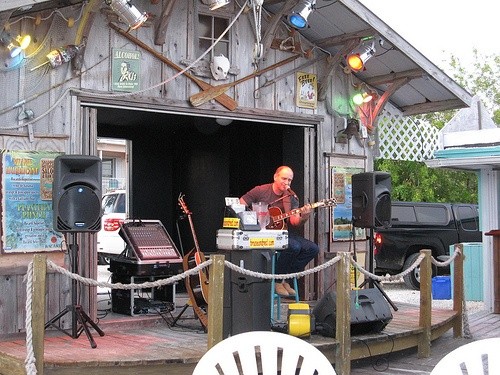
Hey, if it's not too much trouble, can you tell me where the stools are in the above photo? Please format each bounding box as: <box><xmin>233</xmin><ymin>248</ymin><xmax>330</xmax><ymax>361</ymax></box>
<box><xmin>271</xmin><ymin>252</ymin><xmax>299</xmax><ymax>326</ymax></box>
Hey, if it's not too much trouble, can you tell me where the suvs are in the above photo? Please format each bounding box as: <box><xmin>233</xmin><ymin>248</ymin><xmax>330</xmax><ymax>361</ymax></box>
<box><xmin>374</xmin><ymin>202</ymin><xmax>483</xmax><ymax>291</ymax></box>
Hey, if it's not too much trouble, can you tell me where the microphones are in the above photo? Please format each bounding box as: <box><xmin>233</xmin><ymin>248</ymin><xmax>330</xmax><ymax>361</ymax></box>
<box><xmin>285</xmin><ymin>185</ymin><xmax>301</xmax><ymax>201</ymax></box>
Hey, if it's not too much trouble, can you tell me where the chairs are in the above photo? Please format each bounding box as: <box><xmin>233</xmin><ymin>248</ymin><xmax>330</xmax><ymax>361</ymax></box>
<box><xmin>192</xmin><ymin>331</ymin><xmax>337</xmax><ymax>375</ymax></box>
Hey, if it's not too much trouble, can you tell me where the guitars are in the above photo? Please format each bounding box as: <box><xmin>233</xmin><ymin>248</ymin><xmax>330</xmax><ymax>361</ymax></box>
<box><xmin>265</xmin><ymin>198</ymin><xmax>337</xmax><ymax>230</ymax></box>
<box><xmin>177</xmin><ymin>193</ymin><xmax>209</xmax><ymax>307</ymax></box>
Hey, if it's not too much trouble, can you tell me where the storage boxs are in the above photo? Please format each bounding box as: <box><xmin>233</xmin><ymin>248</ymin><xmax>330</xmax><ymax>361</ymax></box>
<box><xmin>431</xmin><ymin>278</ymin><xmax>451</xmax><ymax>300</ymax></box>
<box><xmin>224</xmin><ymin>206</ymin><xmax>261</xmax><ymax>231</ymax></box>
<box><xmin>216</xmin><ymin>229</ymin><xmax>289</xmax><ymax>250</ymax></box>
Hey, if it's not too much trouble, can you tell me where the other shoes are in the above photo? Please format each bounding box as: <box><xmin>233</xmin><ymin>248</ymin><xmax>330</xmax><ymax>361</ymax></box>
<box><xmin>283</xmin><ymin>282</ymin><xmax>296</xmax><ymax>295</ymax></box>
<box><xmin>275</xmin><ymin>282</ymin><xmax>290</xmax><ymax>297</ymax></box>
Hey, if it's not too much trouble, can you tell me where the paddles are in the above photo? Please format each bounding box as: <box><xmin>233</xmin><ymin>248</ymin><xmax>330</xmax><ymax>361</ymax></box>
<box><xmin>189</xmin><ymin>53</ymin><xmax>304</xmax><ymax>106</ymax></box>
<box><xmin>108</xmin><ymin>22</ymin><xmax>237</xmax><ymax>110</ymax></box>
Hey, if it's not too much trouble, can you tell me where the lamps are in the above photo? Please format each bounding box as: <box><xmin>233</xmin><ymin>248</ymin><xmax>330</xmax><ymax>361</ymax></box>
<box><xmin>346</xmin><ymin>36</ymin><xmax>392</xmax><ymax>72</ymax></box>
<box><xmin>286</xmin><ymin>0</ymin><xmax>317</xmax><ymax>29</ymax></box>
<box><xmin>353</xmin><ymin>83</ymin><xmax>379</xmax><ymax>104</ymax></box>
<box><xmin>206</xmin><ymin>0</ymin><xmax>230</xmax><ymax>11</ymax></box>
<box><xmin>105</xmin><ymin>0</ymin><xmax>150</xmax><ymax>30</ymax></box>
<box><xmin>0</xmin><ymin>32</ymin><xmax>30</xmax><ymax>58</ymax></box>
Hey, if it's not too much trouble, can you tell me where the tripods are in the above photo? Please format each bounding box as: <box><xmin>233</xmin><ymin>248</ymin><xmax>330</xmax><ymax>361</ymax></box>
<box><xmin>358</xmin><ymin>227</ymin><xmax>398</xmax><ymax>312</ymax></box>
<box><xmin>25</xmin><ymin>233</ymin><xmax>106</xmax><ymax>349</ymax></box>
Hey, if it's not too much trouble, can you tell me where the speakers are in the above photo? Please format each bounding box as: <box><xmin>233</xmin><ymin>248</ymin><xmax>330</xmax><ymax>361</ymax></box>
<box><xmin>52</xmin><ymin>153</ymin><xmax>103</xmax><ymax>233</ymax></box>
<box><xmin>350</xmin><ymin>170</ymin><xmax>393</xmax><ymax>230</ymax></box>
<box><xmin>312</xmin><ymin>287</ymin><xmax>394</xmax><ymax>335</ymax></box>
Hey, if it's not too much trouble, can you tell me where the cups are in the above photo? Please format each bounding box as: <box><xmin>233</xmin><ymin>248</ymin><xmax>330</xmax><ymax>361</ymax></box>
<box><xmin>251</xmin><ymin>202</ymin><xmax>270</xmax><ymax>231</ymax></box>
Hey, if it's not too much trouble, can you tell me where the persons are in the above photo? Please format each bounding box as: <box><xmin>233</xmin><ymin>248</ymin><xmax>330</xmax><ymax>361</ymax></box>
<box><xmin>240</xmin><ymin>166</ymin><xmax>320</xmax><ymax>296</ymax></box>
